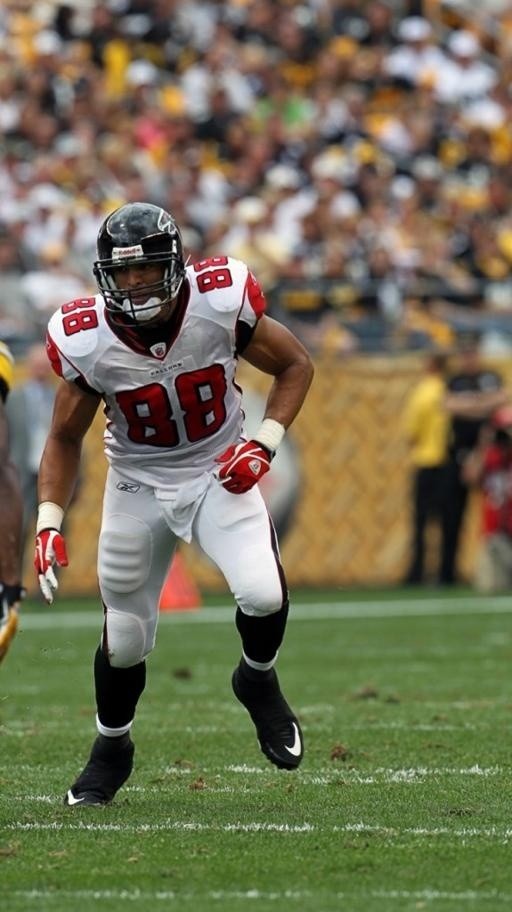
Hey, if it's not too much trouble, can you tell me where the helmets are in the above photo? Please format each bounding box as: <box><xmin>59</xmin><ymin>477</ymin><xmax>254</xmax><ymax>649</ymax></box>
<box><xmin>93</xmin><ymin>200</ymin><xmax>187</xmax><ymax>330</ymax></box>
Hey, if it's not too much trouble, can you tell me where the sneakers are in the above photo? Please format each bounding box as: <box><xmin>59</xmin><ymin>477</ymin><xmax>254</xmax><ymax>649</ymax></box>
<box><xmin>63</xmin><ymin>734</ymin><xmax>136</xmax><ymax>810</ymax></box>
<box><xmin>231</xmin><ymin>659</ymin><xmax>304</xmax><ymax>772</ymax></box>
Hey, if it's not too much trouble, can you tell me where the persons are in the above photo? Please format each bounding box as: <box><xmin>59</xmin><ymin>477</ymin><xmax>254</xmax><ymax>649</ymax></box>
<box><xmin>32</xmin><ymin>200</ymin><xmax>315</xmax><ymax>806</ymax></box>
<box><xmin>0</xmin><ymin>1</ymin><xmax>510</xmax><ymax>660</ymax></box>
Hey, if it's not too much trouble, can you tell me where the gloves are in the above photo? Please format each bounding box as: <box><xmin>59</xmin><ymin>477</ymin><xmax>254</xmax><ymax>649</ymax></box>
<box><xmin>31</xmin><ymin>527</ymin><xmax>70</xmax><ymax>606</ymax></box>
<box><xmin>209</xmin><ymin>439</ymin><xmax>272</xmax><ymax>495</ymax></box>
